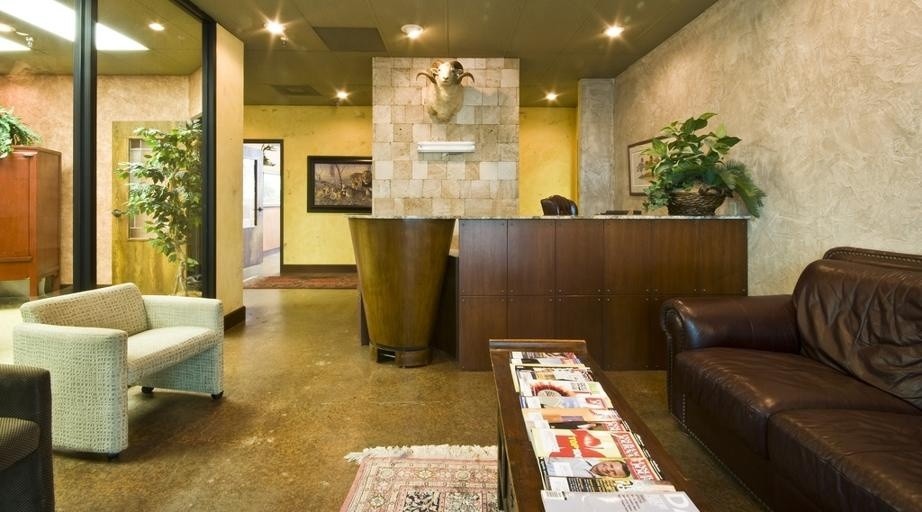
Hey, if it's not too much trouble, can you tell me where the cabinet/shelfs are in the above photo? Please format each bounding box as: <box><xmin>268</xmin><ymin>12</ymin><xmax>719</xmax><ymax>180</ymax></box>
<box><xmin>1</xmin><ymin>144</ymin><xmax>62</xmax><ymax>303</ymax></box>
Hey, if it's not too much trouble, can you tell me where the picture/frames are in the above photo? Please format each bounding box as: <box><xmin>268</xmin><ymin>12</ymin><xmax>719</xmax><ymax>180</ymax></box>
<box><xmin>306</xmin><ymin>155</ymin><xmax>373</xmax><ymax>215</ymax></box>
<box><xmin>628</xmin><ymin>135</ymin><xmax>679</xmax><ymax>197</ymax></box>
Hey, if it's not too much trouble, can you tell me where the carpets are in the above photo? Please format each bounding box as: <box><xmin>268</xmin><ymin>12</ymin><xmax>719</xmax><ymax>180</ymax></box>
<box><xmin>335</xmin><ymin>443</ymin><xmax>500</xmax><ymax>512</ymax></box>
<box><xmin>243</xmin><ymin>273</ymin><xmax>359</xmax><ymax>290</ymax></box>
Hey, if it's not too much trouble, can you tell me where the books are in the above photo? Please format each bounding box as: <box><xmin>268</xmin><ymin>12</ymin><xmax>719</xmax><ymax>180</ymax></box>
<box><xmin>505</xmin><ymin>347</ymin><xmax>700</xmax><ymax>511</ymax></box>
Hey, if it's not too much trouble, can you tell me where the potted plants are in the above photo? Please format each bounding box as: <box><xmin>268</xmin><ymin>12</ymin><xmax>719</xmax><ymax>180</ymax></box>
<box><xmin>640</xmin><ymin>113</ymin><xmax>767</xmax><ymax>218</ymax></box>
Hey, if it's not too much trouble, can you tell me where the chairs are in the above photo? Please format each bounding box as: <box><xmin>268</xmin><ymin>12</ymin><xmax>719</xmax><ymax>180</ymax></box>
<box><xmin>541</xmin><ymin>194</ymin><xmax>578</xmax><ymax>216</ymax></box>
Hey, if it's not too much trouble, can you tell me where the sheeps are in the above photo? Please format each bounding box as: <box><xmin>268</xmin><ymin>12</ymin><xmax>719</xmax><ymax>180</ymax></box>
<box><xmin>414</xmin><ymin>57</ymin><xmax>473</xmax><ymax>125</ymax></box>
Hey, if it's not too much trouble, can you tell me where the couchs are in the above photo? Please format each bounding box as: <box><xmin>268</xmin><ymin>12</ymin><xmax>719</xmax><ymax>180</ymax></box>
<box><xmin>657</xmin><ymin>247</ymin><xmax>921</xmax><ymax>512</ymax></box>
<box><xmin>12</xmin><ymin>281</ymin><xmax>225</xmax><ymax>457</ymax></box>
<box><xmin>0</xmin><ymin>361</ymin><xmax>55</xmax><ymax>512</ymax></box>
